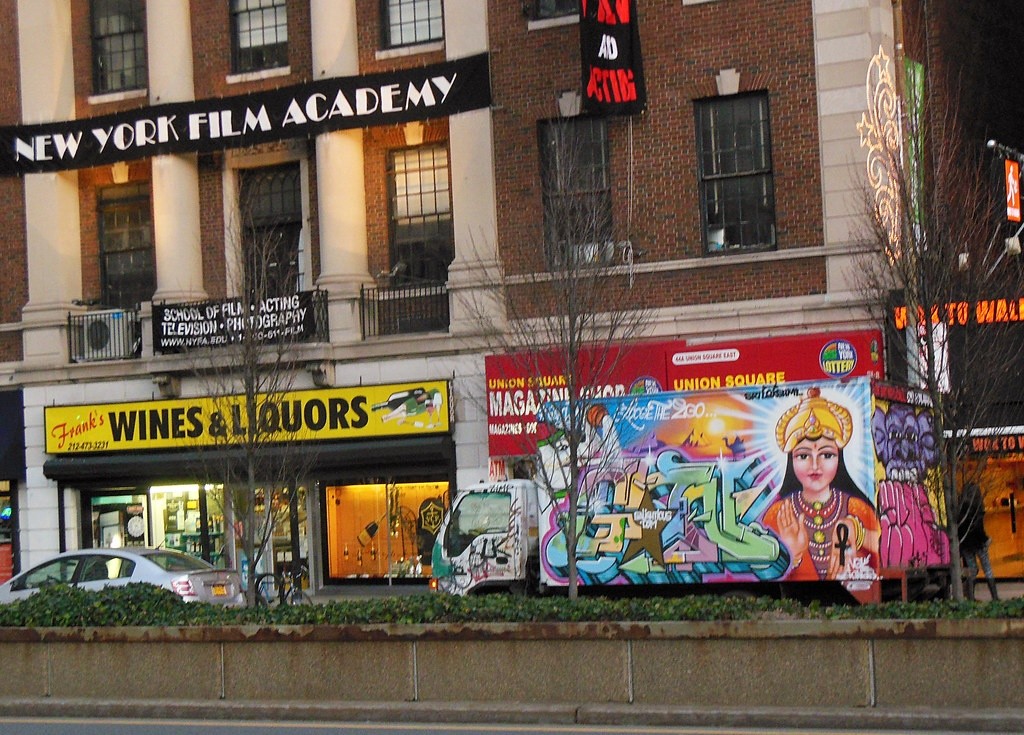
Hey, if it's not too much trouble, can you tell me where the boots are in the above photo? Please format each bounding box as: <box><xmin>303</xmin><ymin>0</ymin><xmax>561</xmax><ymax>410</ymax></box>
<box><xmin>989</xmin><ymin>579</ymin><xmax>998</xmax><ymax>600</ymax></box>
<box><xmin>968</xmin><ymin>580</ymin><xmax>975</xmax><ymax>601</ymax></box>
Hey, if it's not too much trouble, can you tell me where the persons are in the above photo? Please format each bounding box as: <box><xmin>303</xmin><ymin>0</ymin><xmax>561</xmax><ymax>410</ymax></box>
<box><xmin>956</xmin><ymin>482</ymin><xmax>1000</xmax><ymax>602</ymax></box>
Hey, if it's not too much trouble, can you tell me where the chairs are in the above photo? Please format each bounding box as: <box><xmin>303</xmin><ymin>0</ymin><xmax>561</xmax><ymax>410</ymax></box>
<box><xmin>125</xmin><ymin>565</ymin><xmax>134</xmax><ymax>577</ymax></box>
<box><xmin>92</xmin><ymin>562</ymin><xmax>108</xmax><ymax>579</ymax></box>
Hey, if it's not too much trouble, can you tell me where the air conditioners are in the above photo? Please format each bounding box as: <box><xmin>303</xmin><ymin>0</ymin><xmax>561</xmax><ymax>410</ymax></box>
<box><xmin>84</xmin><ymin>309</ymin><xmax>131</xmax><ymax>359</ymax></box>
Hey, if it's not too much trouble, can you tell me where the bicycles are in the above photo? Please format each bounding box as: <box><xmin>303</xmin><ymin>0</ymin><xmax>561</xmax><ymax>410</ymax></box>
<box><xmin>253</xmin><ymin>562</ymin><xmax>316</xmax><ymax>608</ymax></box>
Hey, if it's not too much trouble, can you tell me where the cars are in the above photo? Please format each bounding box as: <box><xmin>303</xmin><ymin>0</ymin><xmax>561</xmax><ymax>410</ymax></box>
<box><xmin>2</xmin><ymin>546</ymin><xmax>248</xmax><ymax>613</ymax></box>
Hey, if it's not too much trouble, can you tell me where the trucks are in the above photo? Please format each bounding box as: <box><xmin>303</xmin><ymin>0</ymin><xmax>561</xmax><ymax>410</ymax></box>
<box><xmin>429</xmin><ymin>372</ymin><xmax>952</xmax><ymax>608</ymax></box>
